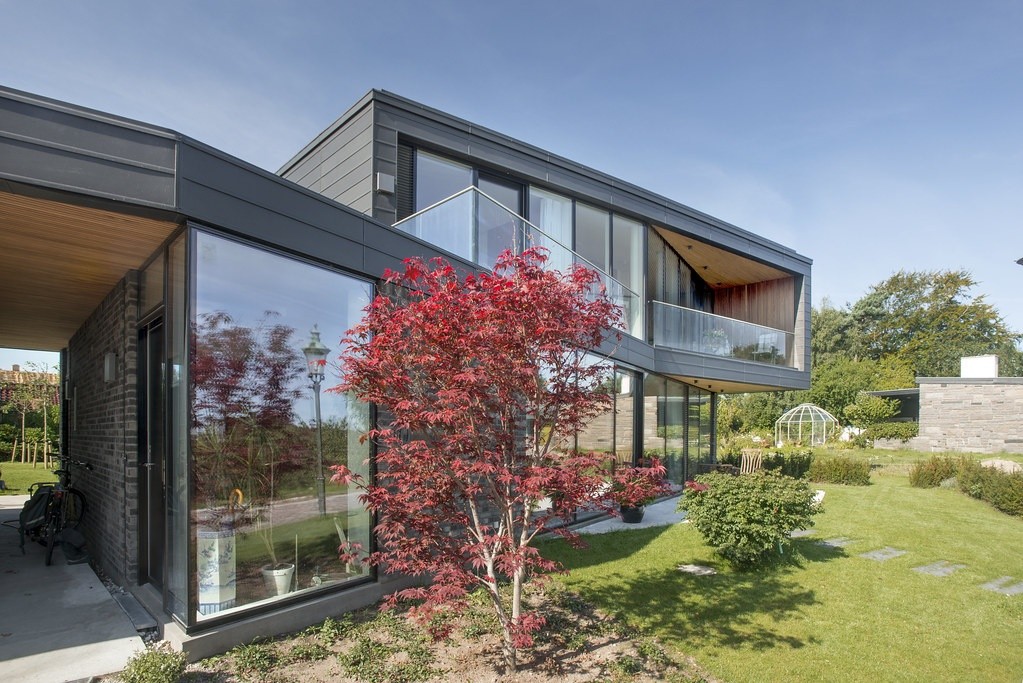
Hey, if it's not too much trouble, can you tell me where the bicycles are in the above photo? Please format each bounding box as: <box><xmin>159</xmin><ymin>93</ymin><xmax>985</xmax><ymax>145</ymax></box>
<box><xmin>31</xmin><ymin>452</ymin><xmax>95</xmax><ymax>566</ymax></box>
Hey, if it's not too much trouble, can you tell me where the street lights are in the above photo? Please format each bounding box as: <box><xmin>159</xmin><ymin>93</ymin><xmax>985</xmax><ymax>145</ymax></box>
<box><xmin>300</xmin><ymin>322</ymin><xmax>332</xmax><ymax>516</ymax></box>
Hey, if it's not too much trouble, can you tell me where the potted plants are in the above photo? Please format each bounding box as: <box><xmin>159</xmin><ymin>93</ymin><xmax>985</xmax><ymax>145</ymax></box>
<box><xmin>192</xmin><ymin>422</ymin><xmax>247</xmax><ymax>614</ymax></box>
<box><xmin>231</xmin><ymin>401</ymin><xmax>295</xmax><ymax>596</ymax></box>
<box><xmin>614</xmin><ymin>474</ymin><xmax>656</xmax><ymax>523</ymax></box>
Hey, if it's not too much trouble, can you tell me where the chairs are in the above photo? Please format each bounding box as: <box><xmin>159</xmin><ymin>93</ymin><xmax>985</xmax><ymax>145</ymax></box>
<box><xmin>751</xmin><ymin>332</ymin><xmax>779</xmax><ymax>367</ymax></box>
